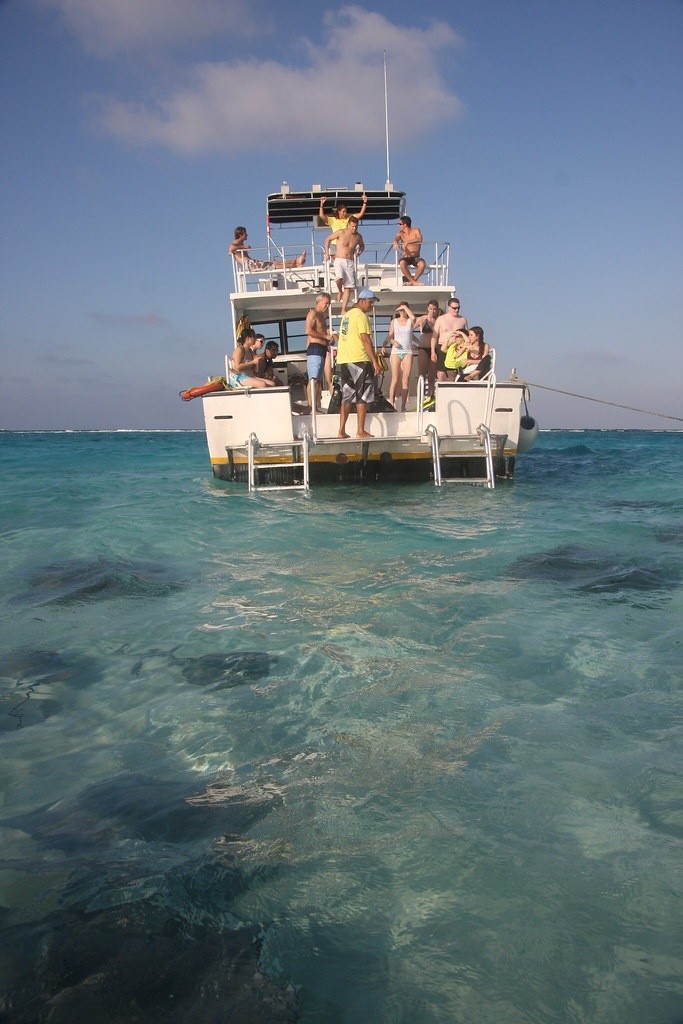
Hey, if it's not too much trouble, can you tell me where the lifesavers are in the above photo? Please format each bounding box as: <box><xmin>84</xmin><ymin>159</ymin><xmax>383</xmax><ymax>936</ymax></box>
<box><xmin>288</xmin><ymin>372</ymin><xmax>312</xmax><ymax>414</ymax></box>
<box><xmin>179</xmin><ymin>380</ymin><xmax>226</xmax><ymax>400</ymax></box>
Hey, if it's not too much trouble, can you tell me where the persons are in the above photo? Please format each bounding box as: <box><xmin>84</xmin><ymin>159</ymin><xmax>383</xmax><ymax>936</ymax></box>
<box><xmin>394</xmin><ymin>216</ymin><xmax>426</xmax><ymax>285</ymax></box>
<box><xmin>382</xmin><ymin>331</ymin><xmax>420</xmax><ymax>402</ymax></box>
<box><xmin>412</xmin><ymin>300</ymin><xmax>441</xmax><ymax>398</ymax></box>
<box><xmin>336</xmin><ymin>289</ymin><xmax>381</xmax><ymax>438</ymax></box>
<box><xmin>229</xmin><ymin>227</ymin><xmax>305</xmax><ymax>272</ymax></box>
<box><xmin>324</xmin><ymin>216</ymin><xmax>364</xmax><ymax>314</ymax></box>
<box><xmin>431</xmin><ymin>298</ymin><xmax>468</xmax><ymax>382</ymax></box>
<box><xmin>231</xmin><ymin>328</ymin><xmax>311</xmax><ymax>414</ymax></box>
<box><xmin>389</xmin><ymin>302</ymin><xmax>416</xmax><ymax>411</ymax></box>
<box><xmin>305</xmin><ymin>292</ymin><xmax>340</xmax><ymax>414</ymax></box>
<box><xmin>441</xmin><ymin>326</ymin><xmax>491</xmax><ymax>382</ymax></box>
<box><xmin>319</xmin><ymin>195</ymin><xmax>367</xmax><ymax>260</ymax></box>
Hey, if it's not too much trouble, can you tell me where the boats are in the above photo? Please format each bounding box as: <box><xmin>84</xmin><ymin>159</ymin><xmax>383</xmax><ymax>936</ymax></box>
<box><xmin>179</xmin><ymin>49</ymin><xmax>538</xmax><ymax>497</ymax></box>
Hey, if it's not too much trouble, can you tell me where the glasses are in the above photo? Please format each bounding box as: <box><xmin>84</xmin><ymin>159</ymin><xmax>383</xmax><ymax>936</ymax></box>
<box><xmin>398</xmin><ymin>223</ymin><xmax>402</xmax><ymax>226</ymax></box>
<box><xmin>256</xmin><ymin>338</ymin><xmax>264</xmax><ymax>343</ymax></box>
<box><xmin>450</xmin><ymin>306</ymin><xmax>460</xmax><ymax>310</ymax></box>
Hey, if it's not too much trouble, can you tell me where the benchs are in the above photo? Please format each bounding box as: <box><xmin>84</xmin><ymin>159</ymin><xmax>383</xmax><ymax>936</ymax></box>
<box><xmin>224</xmin><ymin>355</ymin><xmax>255</xmax><ymax>389</ymax></box>
<box><xmin>453</xmin><ymin>348</ymin><xmax>496</xmax><ymax>381</ymax></box>
<box><xmin>364</xmin><ymin>264</ymin><xmax>446</xmax><ymax>286</ymax></box>
<box><xmin>237</xmin><ymin>265</ymin><xmax>326</xmax><ymax>293</ymax></box>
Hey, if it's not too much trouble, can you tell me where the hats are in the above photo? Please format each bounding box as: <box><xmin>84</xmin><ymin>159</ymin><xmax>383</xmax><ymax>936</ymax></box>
<box><xmin>358</xmin><ymin>289</ymin><xmax>379</xmax><ymax>299</ymax></box>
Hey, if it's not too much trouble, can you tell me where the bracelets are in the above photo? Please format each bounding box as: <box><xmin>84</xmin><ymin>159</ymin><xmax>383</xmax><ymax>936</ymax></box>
<box><xmin>446</xmin><ymin>340</ymin><xmax>449</xmax><ymax>342</ymax></box>
<box><xmin>364</xmin><ymin>202</ymin><xmax>367</xmax><ymax>204</ymax></box>
<box><xmin>320</xmin><ymin>207</ymin><xmax>323</xmax><ymax>208</ymax></box>
